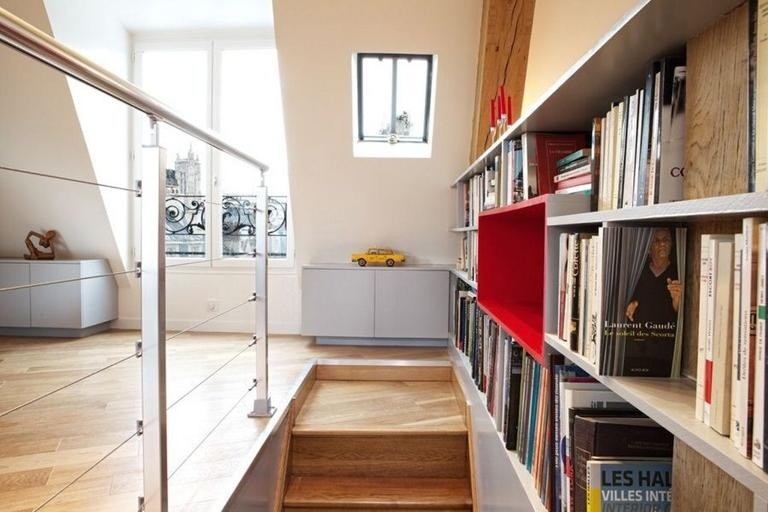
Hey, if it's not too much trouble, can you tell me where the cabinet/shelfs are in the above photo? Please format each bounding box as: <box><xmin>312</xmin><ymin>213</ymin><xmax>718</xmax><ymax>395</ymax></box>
<box><xmin>298</xmin><ymin>262</ymin><xmax>456</xmax><ymax>347</ymax></box>
<box><xmin>444</xmin><ymin>0</ymin><xmax>766</xmax><ymax>512</ymax></box>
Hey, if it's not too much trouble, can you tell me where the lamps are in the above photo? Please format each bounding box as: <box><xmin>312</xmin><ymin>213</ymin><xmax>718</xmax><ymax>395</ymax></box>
<box><xmin>387</xmin><ymin>135</ymin><xmax>398</xmax><ymax>144</ymax></box>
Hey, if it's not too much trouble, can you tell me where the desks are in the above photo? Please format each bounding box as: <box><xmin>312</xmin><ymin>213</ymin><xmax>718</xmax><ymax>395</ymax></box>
<box><xmin>0</xmin><ymin>259</ymin><xmax>119</xmax><ymax>338</ymax></box>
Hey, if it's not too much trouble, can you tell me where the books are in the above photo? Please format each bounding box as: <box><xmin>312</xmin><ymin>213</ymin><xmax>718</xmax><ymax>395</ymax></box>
<box><xmin>461</xmin><ymin>131</ymin><xmax>591</xmax><ymax>282</ymax></box>
<box><xmin>455</xmin><ymin>276</ymin><xmax>553</xmax><ymax>511</ymax></box>
<box><xmin>547</xmin><ymin>353</ymin><xmax>674</xmax><ymax>512</ymax></box>
<box><xmin>590</xmin><ymin>57</ymin><xmax>687</xmax><ymax>212</ymax></box>
<box><xmin>557</xmin><ymin>221</ymin><xmax>686</xmax><ymax>376</ymax></box>
<box><xmin>696</xmin><ymin>216</ymin><xmax>768</xmax><ymax>473</ymax></box>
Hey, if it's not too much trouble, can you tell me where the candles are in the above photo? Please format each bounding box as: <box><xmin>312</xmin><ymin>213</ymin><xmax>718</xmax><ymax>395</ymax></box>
<box><xmin>489</xmin><ymin>85</ymin><xmax>512</xmax><ymax>128</ymax></box>
